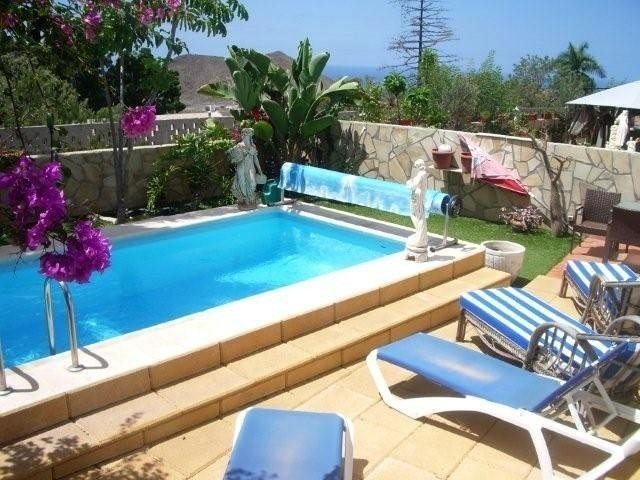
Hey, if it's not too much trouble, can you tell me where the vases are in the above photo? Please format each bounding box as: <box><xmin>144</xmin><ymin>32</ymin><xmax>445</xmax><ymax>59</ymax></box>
<box><xmin>480</xmin><ymin>236</ymin><xmax>526</xmax><ymax>282</ymax></box>
<box><xmin>458</xmin><ymin>152</ymin><xmax>472</xmax><ymax>174</ymax></box>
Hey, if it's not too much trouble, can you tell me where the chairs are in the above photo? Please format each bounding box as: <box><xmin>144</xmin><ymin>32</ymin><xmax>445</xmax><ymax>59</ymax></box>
<box><xmin>363</xmin><ymin>327</ymin><xmax>638</xmax><ymax>480</ymax></box>
<box><xmin>556</xmin><ymin>258</ymin><xmax>640</xmax><ymax>333</ymax></box>
<box><xmin>568</xmin><ymin>188</ymin><xmax>622</xmax><ymax>256</ymax></box>
<box><xmin>452</xmin><ymin>284</ymin><xmax>640</xmax><ymax>402</ymax></box>
<box><xmin>218</xmin><ymin>403</ymin><xmax>358</xmax><ymax>479</ymax></box>
<box><xmin>603</xmin><ymin>202</ymin><xmax>639</xmax><ymax>268</ymax></box>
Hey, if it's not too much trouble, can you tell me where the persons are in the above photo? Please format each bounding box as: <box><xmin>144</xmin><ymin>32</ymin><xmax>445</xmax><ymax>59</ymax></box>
<box><xmin>406</xmin><ymin>159</ymin><xmax>432</xmax><ymax>247</ymax></box>
<box><xmin>227</xmin><ymin>128</ymin><xmax>261</xmax><ymax>204</ymax></box>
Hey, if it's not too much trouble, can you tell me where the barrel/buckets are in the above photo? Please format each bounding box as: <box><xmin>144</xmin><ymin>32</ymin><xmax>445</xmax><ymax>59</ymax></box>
<box><xmin>262</xmin><ymin>176</ymin><xmax>281</xmax><ymax>205</ymax></box>
<box><xmin>461</xmin><ymin>152</ymin><xmax>472</xmax><ymax>172</ymax></box>
<box><xmin>481</xmin><ymin>240</ymin><xmax>525</xmax><ymax>284</ymax></box>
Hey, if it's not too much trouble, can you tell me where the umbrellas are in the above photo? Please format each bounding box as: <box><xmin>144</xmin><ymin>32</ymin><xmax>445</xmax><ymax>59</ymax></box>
<box><xmin>565</xmin><ymin>80</ymin><xmax>640</xmax><ymax>110</ymax></box>
